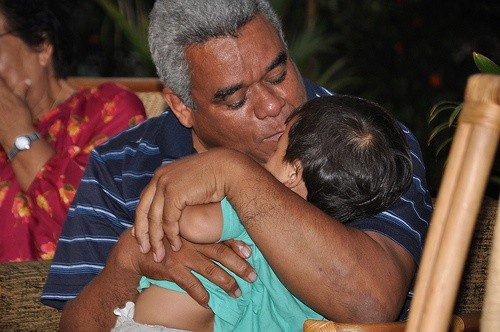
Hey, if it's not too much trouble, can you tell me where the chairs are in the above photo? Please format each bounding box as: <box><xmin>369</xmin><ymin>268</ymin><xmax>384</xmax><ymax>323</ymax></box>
<box><xmin>0</xmin><ymin>199</ymin><xmax>500</xmax><ymax>332</ymax></box>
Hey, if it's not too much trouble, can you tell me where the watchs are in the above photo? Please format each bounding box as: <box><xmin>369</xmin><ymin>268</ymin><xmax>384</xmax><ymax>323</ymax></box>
<box><xmin>8</xmin><ymin>132</ymin><xmax>40</xmax><ymax>160</ymax></box>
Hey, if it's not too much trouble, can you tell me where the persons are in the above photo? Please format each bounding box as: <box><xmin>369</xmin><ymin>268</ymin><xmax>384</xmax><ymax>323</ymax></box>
<box><xmin>38</xmin><ymin>0</ymin><xmax>432</xmax><ymax>332</ymax></box>
<box><xmin>111</xmin><ymin>94</ymin><xmax>415</xmax><ymax>331</ymax></box>
<box><xmin>0</xmin><ymin>0</ymin><xmax>146</xmax><ymax>263</ymax></box>
